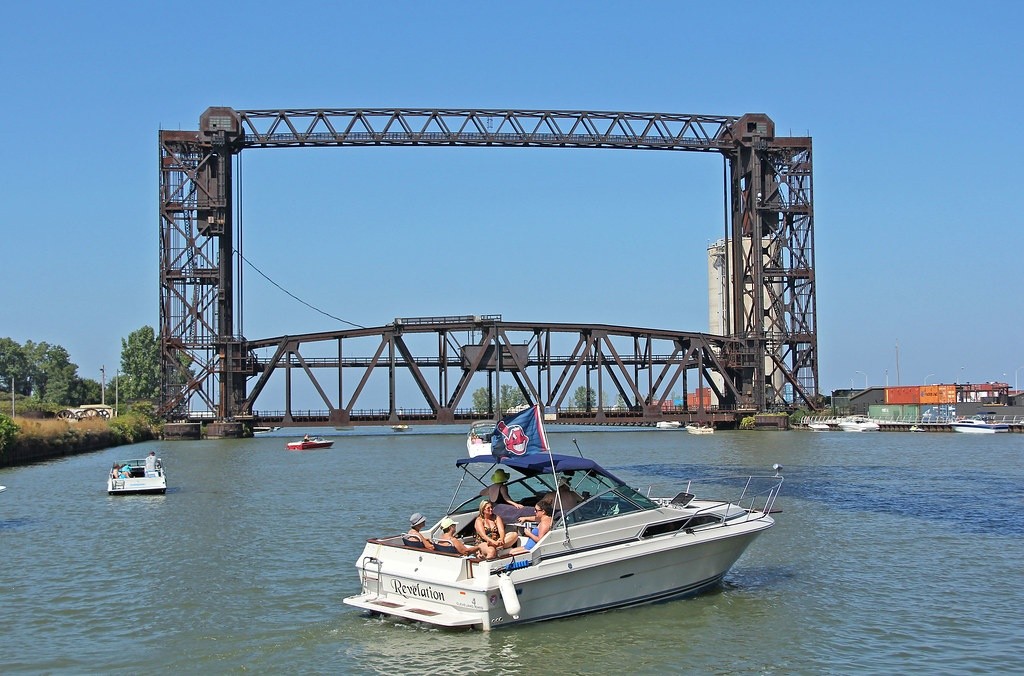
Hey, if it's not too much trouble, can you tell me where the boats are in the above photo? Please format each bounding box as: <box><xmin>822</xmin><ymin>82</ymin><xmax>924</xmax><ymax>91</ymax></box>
<box><xmin>389</xmin><ymin>424</ymin><xmax>413</xmax><ymax>432</ymax></box>
<box><xmin>808</xmin><ymin>424</ymin><xmax>830</xmax><ymax>432</ymax></box>
<box><xmin>837</xmin><ymin>414</ymin><xmax>881</xmax><ymax>433</ymax></box>
<box><xmin>947</xmin><ymin>419</ymin><xmax>1010</xmax><ymax>433</ymax></box>
<box><xmin>656</xmin><ymin>421</ymin><xmax>682</xmax><ymax>429</ymax></box>
<box><xmin>341</xmin><ymin>395</ymin><xmax>785</xmax><ymax>630</ymax></box>
<box><xmin>909</xmin><ymin>424</ymin><xmax>926</xmax><ymax>432</ymax></box>
<box><xmin>106</xmin><ymin>458</ymin><xmax>167</xmax><ymax>495</ymax></box>
<box><xmin>287</xmin><ymin>436</ymin><xmax>335</xmax><ymax>450</ymax></box>
<box><xmin>466</xmin><ymin>420</ymin><xmax>499</xmax><ymax>459</ymax></box>
<box><xmin>684</xmin><ymin>425</ymin><xmax>715</xmax><ymax>435</ymax></box>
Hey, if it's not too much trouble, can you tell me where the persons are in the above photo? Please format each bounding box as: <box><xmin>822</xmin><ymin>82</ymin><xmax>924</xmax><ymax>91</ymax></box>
<box><xmin>408</xmin><ymin>513</ymin><xmax>434</xmax><ymax>548</ymax></box>
<box><xmin>145</xmin><ymin>451</ymin><xmax>159</xmax><ymax>472</ymax></box>
<box><xmin>304</xmin><ymin>434</ymin><xmax>311</xmax><ymax>442</ymax></box>
<box><xmin>480</xmin><ymin>469</ymin><xmax>524</xmax><ymax>509</ymax></box>
<box><xmin>439</xmin><ymin>518</ymin><xmax>478</xmax><ymax>554</ymax></box>
<box><xmin>475</xmin><ymin>500</ymin><xmax>518</xmax><ymax>558</ymax></box>
<box><xmin>509</xmin><ymin>501</ymin><xmax>553</xmax><ymax>554</ymax></box>
<box><xmin>542</xmin><ymin>476</ymin><xmax>582</xmax><ymax>514</ymax></box>
<box><xmin>113</xmin><ymin>464</ymin><xmax>130</xmax><ymax>478</ymax></box>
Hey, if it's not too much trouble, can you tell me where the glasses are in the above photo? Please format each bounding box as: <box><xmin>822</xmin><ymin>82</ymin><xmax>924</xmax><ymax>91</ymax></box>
<box><xmin>484</xmin><ymin>506</ymin><xmax>493</xmax><ymax>510</ymax></box>
<box><xmin>534</xmin><ymin>509</ymin><xmax>543</xmax><ymax>513</ymax></box>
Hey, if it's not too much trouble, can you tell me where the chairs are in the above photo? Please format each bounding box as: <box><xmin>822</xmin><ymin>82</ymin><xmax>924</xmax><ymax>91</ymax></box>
<box><xmin>401</xmin><ymin>532</ymin><xmax>426</xmax><ymax>548</ymax></box>
<box><xmin>147</xmin><ymin>471</ymin><xmax>159</xmax><ymax>478</ymax></box>
<box><xmin>432</xmin><ymin>538</ymin><xmax>459</xmax><ymax>554</ymax></box>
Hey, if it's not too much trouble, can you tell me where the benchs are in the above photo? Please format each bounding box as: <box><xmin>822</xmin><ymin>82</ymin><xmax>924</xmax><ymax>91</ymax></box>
<box><xmin>492</xmin><ymin>504</ymin><xmax>569</xmax><ymax>523</ymax></box>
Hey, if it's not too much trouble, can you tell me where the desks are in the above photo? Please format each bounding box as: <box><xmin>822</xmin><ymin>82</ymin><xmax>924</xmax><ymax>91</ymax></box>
<box><xmin>505</xmin><ymin>521</ymin><xmax>540</xmax><ymax>536</ymax></box>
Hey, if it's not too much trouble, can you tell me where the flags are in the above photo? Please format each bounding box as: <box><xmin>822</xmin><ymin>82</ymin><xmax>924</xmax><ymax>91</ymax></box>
<box><xmin>491</xmin><ymin>405</ymin><xmax>547</xmax><ymax>460</ymax></box>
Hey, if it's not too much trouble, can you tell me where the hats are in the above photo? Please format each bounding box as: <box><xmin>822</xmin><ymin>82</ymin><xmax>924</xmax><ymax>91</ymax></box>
<box><xmin>409</xmin><ymin>513</ymin><xmax>427</xmax><ymax>526</ymax></box>
<box><xmin>441</xmin><ymin>518</ymin><xmax>459</xmax><ymax>529</ymax></box>
<box><xmin>556</xmin><ymin>476</ymin><xmax>572</xmax><ymax>487</ymax></box>
<box><xmin>491</xmin><ymin>469</ymin><xmax>510</xmax><ymax>483</ymax></box>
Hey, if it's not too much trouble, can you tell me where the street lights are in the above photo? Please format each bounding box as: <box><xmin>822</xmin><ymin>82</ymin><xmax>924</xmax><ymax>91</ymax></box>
<box><xmin>1015</xmin><ymin>366</ymin><xmax>1024</xmax><ymax>395</ymax></box>
<box><xmin>855</xmin><ymin>371</ymin><xmax>868</xmax><ymax>388</ymax></box>
<box><xmin>924</xmin><ymin>373</ymin><xmax>936</xmax><ymax>386</ymax></box>
